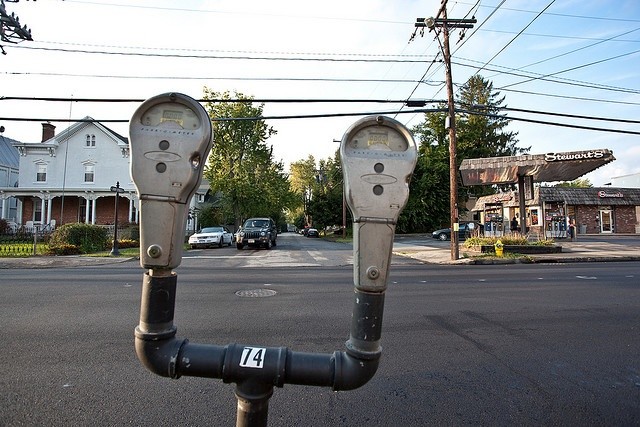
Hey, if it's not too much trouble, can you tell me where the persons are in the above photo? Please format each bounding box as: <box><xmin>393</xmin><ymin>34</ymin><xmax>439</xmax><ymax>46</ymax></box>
<box><xmin>511</xmin><ymin>217</ymin><xmax>518</xmax><ymax>230</ymax></box>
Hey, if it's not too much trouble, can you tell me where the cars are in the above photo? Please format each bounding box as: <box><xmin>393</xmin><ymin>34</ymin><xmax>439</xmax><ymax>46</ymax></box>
<box><xmin>432</xmin><ymin>220</ymin><xmax>484</xmax><ymax>241</ymax></box>
<box><xmin>306</xmin><ymin>228</ymin><xmax>320</xmax><ymax>238</ymax></box>
<box><xmin>187</xmin><ymin>226</ymin><xmax>234</xmax><ymax>250</ymax></box>
<box><xmin>235</xmin><ymin>217</ymin><xmax>277</xmax><ymax>250</ymax></box>
